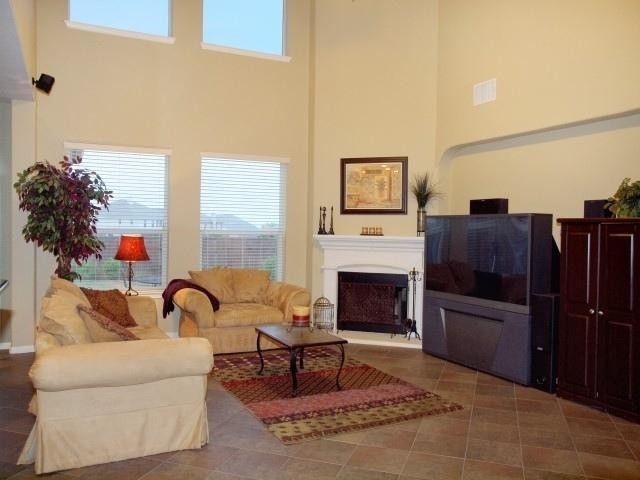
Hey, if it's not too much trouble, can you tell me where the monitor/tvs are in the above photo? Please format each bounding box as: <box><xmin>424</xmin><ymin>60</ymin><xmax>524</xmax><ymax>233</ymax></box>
<box><xmin>423</xmin><ymin>213</ymin><xmax>561</xmax><ymax>314</ymax></box>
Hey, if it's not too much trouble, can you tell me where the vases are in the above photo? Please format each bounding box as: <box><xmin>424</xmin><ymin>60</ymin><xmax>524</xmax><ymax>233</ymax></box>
<box><xmin>416</xmin><ymin>206</ymin><xmax>427</xmax><ymax>236</ymax></box>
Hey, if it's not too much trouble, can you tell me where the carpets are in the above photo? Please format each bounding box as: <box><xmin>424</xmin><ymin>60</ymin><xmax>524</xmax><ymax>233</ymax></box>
<box><xmin>209</xmin><ymin>345</ymin><xmax>464</xmax><ymax>445</ymax></box>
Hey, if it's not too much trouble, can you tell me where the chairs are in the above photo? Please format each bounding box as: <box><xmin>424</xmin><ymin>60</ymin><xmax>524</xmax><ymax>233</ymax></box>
<box><xmin>171</xmin><ymin>274</ymin><xmax>310</xmax><ymax>356</ymax></box>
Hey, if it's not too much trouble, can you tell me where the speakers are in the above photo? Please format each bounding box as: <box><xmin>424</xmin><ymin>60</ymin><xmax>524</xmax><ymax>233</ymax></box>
<box><xmin>470</xmin><ymin>199</ymin><xmax>509</xmax><ymax>214</ymax></box>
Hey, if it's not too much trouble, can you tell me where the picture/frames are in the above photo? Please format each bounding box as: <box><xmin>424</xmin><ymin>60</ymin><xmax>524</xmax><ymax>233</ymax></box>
<box><xmin>339</xmin><ymin>157</ymin><xmax>407</xmax><ymax>214</ymax></box>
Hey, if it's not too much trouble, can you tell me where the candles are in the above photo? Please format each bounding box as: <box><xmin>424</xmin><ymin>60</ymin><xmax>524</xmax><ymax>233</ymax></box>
<box><xmin>319</xmin><ymin>206</ymin><xmax>333</xmax><ymax>230</ymax></box>
<box><xmin>362</xmin><ymin>227</ymin><xmax>382</xmax><ymax>234</ymax></box>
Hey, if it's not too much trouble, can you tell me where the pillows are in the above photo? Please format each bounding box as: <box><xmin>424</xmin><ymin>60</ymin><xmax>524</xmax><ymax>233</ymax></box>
<box><xmin>188</xmin><ymin>267</ymin><xmax>271</xmax><ymax>303</ymax></box>
<box><xmin>36</xmin><ymin>274</ymin><xmax>142</xmax><ymax>346</ymax></box>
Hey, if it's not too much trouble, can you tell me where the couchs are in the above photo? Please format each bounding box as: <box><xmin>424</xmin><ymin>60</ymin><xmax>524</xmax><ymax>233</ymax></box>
<box><xmin>16</xmin><ymin>295</ymin><xmax>214</xmax><ymax>475</ymax></box>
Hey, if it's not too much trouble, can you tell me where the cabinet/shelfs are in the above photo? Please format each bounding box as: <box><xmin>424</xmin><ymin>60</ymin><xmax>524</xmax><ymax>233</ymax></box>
<box><xmin>556</xmin><ymin>217</ymin><xmax>637</xmax><ymax>424</ymax></box>
<box><xmin>422</xmin><ymin>296</ymin><xmax>532</xmax><ymax>385</ymax></box>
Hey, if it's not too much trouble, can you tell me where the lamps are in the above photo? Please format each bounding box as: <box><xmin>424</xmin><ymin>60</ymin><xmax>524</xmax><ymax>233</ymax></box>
<box><xmin>114</xmin><ymin>233</ymin><xmax>149</xmax><ymax>294</ymax></box>
